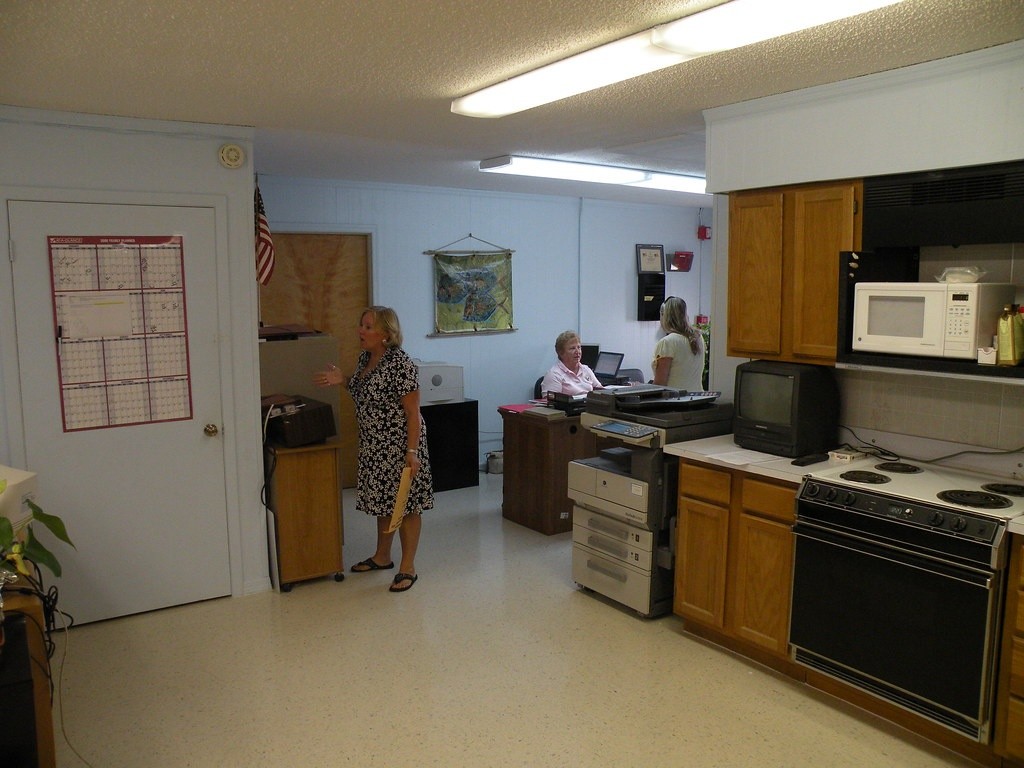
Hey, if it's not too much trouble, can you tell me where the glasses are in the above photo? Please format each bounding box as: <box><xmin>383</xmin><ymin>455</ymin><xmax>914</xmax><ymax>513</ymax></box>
<box><xmin>664</xmin><ymin>295</ymin><xmax>675</xmax><ymax>303</ymax></box>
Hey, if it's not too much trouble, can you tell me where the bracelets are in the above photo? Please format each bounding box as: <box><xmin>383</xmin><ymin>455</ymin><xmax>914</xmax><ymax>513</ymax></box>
<box><xmin>407</xmin><ymin>449</ymin><xmax>418</xmax><ymax>454</ymax></box>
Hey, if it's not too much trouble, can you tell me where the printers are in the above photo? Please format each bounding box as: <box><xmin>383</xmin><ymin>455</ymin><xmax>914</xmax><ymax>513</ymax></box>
<box><xmin>566</xmin><ymin>385</ymin><xmax>733</xmax><ymax>617</ymax></box>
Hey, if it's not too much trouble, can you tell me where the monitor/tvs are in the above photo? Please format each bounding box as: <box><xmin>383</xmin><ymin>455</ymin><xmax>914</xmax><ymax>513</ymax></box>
<box><xmin>580</xmin><ymin>344</ymin><xmax>624</xmax><ymax>379</ymax></box>
<box><xmin>734</xmin><ymin>358</ymin><xmax>841</xmax><ymax>459</ymax></box>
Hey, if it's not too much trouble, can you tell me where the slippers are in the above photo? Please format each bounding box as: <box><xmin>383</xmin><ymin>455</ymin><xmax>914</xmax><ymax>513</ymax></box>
<box><xmin>351</xmin><ymin>557</ymin><xmax>394</xmax><ymax>572</ymax></box>
<box><xmin>390</xmin><ymin>573</ymin><xmax>417</xmax><ymax>591</ymax></box>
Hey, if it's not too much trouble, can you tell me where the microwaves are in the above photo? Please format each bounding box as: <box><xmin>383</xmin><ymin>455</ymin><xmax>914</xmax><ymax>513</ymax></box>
<box><xmin>852</xmin><ymin>282</ymin><xmax>1019</xmax><ymax>358</ymax></box>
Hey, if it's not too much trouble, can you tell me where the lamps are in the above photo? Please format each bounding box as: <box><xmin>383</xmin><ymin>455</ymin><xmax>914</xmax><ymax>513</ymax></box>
<box><xmin>451</xmin><ymin>0</ymin><xmax>904</xmax><ymax>120</ymax></box>
<box><xmin>478</xmin><ymin>156</ymin><xmax>715</xmax><ymax>195</ymax></box>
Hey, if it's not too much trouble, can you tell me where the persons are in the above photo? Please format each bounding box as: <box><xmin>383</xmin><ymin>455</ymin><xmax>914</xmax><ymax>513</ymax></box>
<box><xmin>650</xmin><ymin>294</ymin><xmax>706</xmax><ymax>392</ymax></box>
<box><xmin>312</xmin><ymin>307</ymin><xmax>435</xmax><ymax>591</ymax></box>
<box><xmin>539</xmin><ymin>331</ymin><xmax>603</xmax><ymax>398</ymax></box>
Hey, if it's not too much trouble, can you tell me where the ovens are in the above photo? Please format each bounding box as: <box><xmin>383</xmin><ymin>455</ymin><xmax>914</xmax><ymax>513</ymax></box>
<box><xmin>788</xmin><ymin>517</ymin><xmax>1005</xmax><ymax>745</ymax></box>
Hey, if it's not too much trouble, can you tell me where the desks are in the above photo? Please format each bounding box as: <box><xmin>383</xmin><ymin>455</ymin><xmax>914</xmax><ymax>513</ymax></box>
<box><xmin>263</xmin><ymin>438</ymin><xmax>347</xmax><ymax>593</ymax></box>
<box><xmin>496</xmin><ymin>406</ymin><xmax>606</xmax><ymax>536</ymax></box>
<box><xmin>420</xmin><ymin>398</ymin><xmax>480</xmax><ymax>493</ymax></box>
<box><xmin>0</xmin><ymin>524</ymin><xmax>58</xmax><ymax>768</ymax></box>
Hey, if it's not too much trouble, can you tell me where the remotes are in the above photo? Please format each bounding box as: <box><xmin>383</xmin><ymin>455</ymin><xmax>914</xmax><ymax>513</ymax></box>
<box><xmin>790</xmin><ymin>452</ymin><xmax>830</xmax><ymax>467</ymax></box>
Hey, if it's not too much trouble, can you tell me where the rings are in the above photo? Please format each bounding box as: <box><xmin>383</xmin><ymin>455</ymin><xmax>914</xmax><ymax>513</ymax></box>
<box><xmin>332</xmin><ymin>366</ymin><xmax>336</xmax><ymax>370</ymax></box>
<box><xmin>327</xmin><ymin>384</ymin><xmax>331</xmax><ymax>387</ymax></box>
<box><xmin>323</xmin><ymin>380</ymin><xmax>326</xmax><ymax>383</ymax></box>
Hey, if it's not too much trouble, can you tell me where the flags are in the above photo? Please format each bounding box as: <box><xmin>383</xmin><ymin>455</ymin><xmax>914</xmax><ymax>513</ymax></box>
<box><xmin>254</xmin><ymin>186</ymin><xmax>275</xmax><ymax>287</ymax></box>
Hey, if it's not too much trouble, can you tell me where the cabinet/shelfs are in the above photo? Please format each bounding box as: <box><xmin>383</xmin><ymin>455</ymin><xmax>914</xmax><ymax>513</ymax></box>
<box><xmin>673</xmin><ymin>455</ymin><xmax>1024</xmax><ymax>768</ymax></box>
<box><xmin>728</xmin><ymin>180</ymin><xmax>864</xmax><ymax>367</ymax></box>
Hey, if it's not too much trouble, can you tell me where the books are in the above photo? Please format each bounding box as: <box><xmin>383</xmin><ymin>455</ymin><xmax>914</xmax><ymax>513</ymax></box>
<box><xmin>524</xmin><ymin>407</ymin><xmax>566</xmax><ymax>419</ymax></box>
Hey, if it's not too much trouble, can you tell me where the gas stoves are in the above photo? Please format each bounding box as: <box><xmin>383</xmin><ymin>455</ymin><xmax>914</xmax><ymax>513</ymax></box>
<box><xmin>797</xmin><ymin>455</ymin><xmax>1024</xmax><ymax>570</ymax></box>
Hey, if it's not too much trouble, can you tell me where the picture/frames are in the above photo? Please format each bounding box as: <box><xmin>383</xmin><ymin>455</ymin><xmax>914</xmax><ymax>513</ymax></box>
<box><xmin>636</xmin><ymin>244</ymin><xmax>665</xmax><ymax>275</ymax></box>
